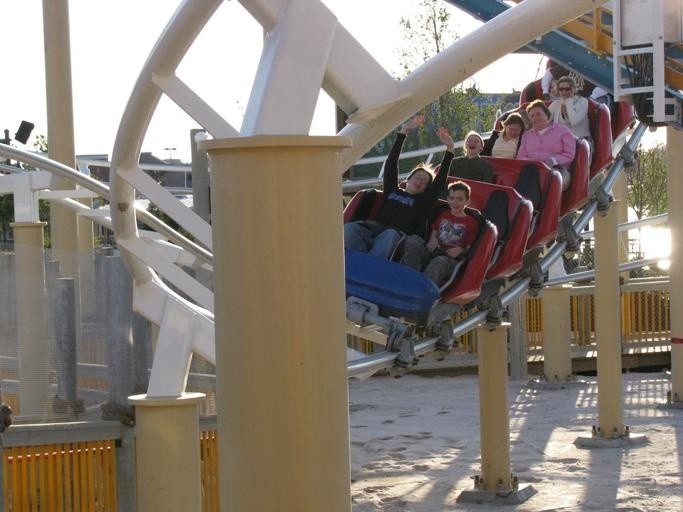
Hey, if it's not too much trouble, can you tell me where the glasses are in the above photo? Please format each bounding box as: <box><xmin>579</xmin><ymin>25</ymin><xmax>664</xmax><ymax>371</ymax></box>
<box><xmin>558</xmin><ymin>87</ymin><xmax>571</xmax><ymax>91</ymax></box>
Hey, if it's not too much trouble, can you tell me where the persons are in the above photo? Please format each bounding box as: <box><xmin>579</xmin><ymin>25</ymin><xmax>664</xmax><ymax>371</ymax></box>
<box><xmin>540</xmin><ymin>59</ymin><xmax>616</xmax><ymax>115</ymax></box>
<box><xmin>546</xmin><ymin>77</ymin><xmax>595</xmax><ymax>167</ymax></box>
<box><xmin>344</xmin><ymin>116</ymin><xmax>453</xmax><ymax>260</ymax></box>
<box><xmin>481</xmin><ymin>112</ymin><xmax>525</xmax><ymax>159</ymax></box>
<box><xmin>448</xmin><ymin>131</ymin><xmax>495</xmax><ymax>185</ymax></box>
<box><xmin>399</xmin><ymin>180</ymin><xmax>475</xmax><ymax>286</ymax></box>
<box><xmin>514</xmin><ymin>99</ymin><xmax>576</xmax><ymax>192</ymax></box>
